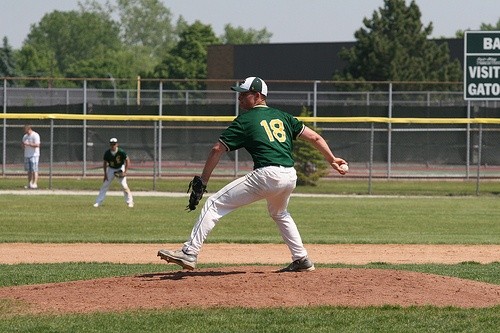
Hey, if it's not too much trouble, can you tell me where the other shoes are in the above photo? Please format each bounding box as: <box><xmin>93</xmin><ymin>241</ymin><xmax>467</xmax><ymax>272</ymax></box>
<box><xmin>30</xmin><ymin>183</ymin><xmax>37</xmax><ymax>189</ymax></box>
<box><xmin>24</xmin><ymin>183</ymin><xmax>30</xmax><ymax>189</ymax></box>
<box><xmin>127</xmin><ymin>202</ymin><xmax>134</xmax><ymax>207</ymax></box>
<box><xmin>93</xmin><ymin>203</ymin><xmax>99</xmax><ymax>208</ymax></box>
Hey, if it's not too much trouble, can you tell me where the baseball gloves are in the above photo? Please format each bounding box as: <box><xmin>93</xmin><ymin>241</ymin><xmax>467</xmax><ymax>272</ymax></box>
<box><xmin>113</xmin><ymin>170</ymin><xmax>124</xmax><ymax>177</ymax></box>
<box><xmin>187</xmin><ymin>176</ymin><xmax>207</xmax><ymax>210</ymax></box>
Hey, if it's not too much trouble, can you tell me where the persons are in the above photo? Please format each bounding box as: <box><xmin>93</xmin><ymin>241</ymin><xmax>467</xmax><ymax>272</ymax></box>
<box><xmin>92</xmin><ymin>138</ymin><xmax>134</xmax><ymax>207</ymax></box>
<box><xmin>21</xmin><ymin>125</ymin><xmax>40</xmax><ymax>189</ymax></box>
<box><xmin>157</xmin><ymin>77</ymin><xmax>348</xmax><ymax>272</ymax></box>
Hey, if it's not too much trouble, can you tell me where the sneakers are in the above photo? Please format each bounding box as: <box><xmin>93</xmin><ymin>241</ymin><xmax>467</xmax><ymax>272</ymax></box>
<box><xmin>276</xmin><ymin>257</ymin><xmax>316</xmax><ymax>274</ymax></box>
<box><xmin>157</xmin><ymin>249</ymin><xmax>197</xmax><ymax>270</ymax></box>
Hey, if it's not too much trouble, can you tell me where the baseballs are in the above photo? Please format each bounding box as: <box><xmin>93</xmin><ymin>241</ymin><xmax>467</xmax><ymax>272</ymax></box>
<box><xmin>340</xmin><ymin>164</ymin><xmax>349</xmax><ymax>173</ymax></box>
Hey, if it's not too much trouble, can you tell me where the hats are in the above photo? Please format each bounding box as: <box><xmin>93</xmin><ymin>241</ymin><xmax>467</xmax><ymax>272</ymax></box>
<box><xmin>109</xmin><ymin>138</ymin><xmax>118</xmax><ymax>144</ymax></box>
<box><xmin>230</xmin><ymin>77</ymin><xmax>268</xmax><ymax>97</ymax></box>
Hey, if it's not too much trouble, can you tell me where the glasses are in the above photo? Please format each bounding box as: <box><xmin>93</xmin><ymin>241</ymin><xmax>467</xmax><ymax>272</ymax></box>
<box><xmin>110</xmin><ymin>144</ymin><xmax>116</xmax><ymax>146</ymax></box>
